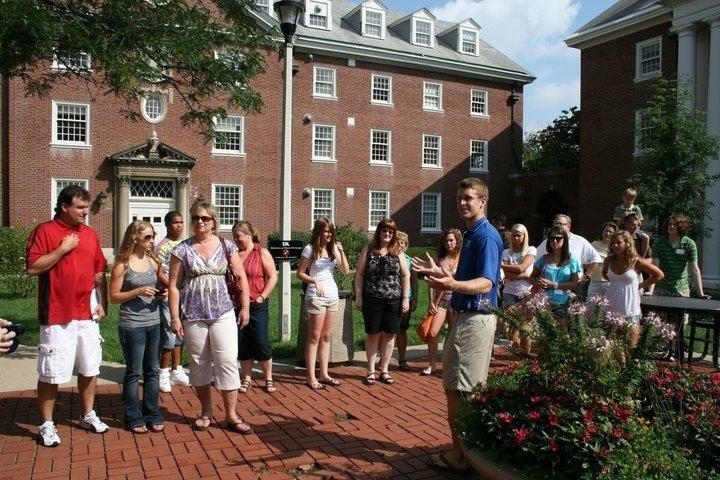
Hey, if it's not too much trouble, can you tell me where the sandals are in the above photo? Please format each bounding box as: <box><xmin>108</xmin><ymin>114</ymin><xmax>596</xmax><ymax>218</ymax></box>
<box><xmin>377</xmin><ymin>359</ymin><xmax>382</xmax><ymax>370</ymax></box>
<box><xmin>379</xmin><ymin>372</ymin><xmax>393</xmax><ymax>384</ymax></box>
<box><xmin>398</xmin><ymin>360</ymin><xmax>411</xmax><ymax>370</ymax></box>
<box><xmin>151</xmin><ymin>424</ymin><xmax>164</xmax><ymax>432</ymax></box>
<box><xmin>265</xmin><ymin>380</ymin><xmax>277</xmax><ymax>393</ymax></box>
<box><xmin>133</xmin><ymin>426</ymin><xmax>149</xmax><ymax>434</ymax></box>
<box><xmin>239</xmin><ymin>380</ymin><xmax>252</xmax><ymax>394</ymax></box>
<box><xmin>366</xmin><ymin>373</ymin><xmax>376</xmax><ymax>385</ymax></box>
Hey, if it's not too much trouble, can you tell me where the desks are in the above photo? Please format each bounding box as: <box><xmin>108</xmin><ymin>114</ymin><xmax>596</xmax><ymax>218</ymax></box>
<box><xmin>638</xmin><ymin>294</ymin><xmax>720</xmax><ymax>372</ymax></box>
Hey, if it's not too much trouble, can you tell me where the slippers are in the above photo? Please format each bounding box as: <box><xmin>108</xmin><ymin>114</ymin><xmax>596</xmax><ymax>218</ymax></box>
<box><xmin>319</xmin><ymin>377</ymin><xmax>341</xmax><ymax>386</ymax></box>
<box><xmin>227</xmin><ymin>420</ymin><xmax>254</xmax><ymax>435</ymax></box>
<box><xmin>307</xmin><ymin>381</ymin><xmax>322</xmax><ymax>389</ymax></box>
<box><xmin>427</xmin><ymin>452</ymin><xmax>471</xmax><ymax>475</ymax></box>
<box><xmin>195</xmin><ymin>416</ymin><xmax>211</xmax><ymax>431</ymax></box>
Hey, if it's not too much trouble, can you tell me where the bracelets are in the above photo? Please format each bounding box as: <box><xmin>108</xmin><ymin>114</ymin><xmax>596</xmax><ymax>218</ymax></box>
<box><xmin>258</xmin><ymin>295</ymin><xmax>265</xmax><ymax>301</ymax></box>
<box><xmin>170</xmin><ymin>318</ymin><xmax>178</xmax><ymax>323</ymax></box>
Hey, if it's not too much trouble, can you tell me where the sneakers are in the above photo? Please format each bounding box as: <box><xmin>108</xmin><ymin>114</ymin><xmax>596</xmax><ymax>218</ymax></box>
<box><xmin>159</xmin><ymin>368</ymin><xmax>172</xmax><ymax>393</ymax></box>
<box><xmin>422</xmin><ymin>365</ymin><xmax>437</xmax><ymax>375</ymax></box>
<box><xmin>38</xmin><ymin>421</ymin><xmax>61</xmax><ymax>447</ymax></box>
<box><xmin>79</xmin><ymin>410</ymin><xmax>109</xmax><ymax>434</ymax></box>
<box><xmin>171</xmin><ymin>366</ymin><xmax>189</xmax><ymax>385</ymax></box>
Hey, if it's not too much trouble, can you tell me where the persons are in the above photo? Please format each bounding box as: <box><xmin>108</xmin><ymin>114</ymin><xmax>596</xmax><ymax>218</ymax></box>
<box><xmin>169</xmin><ymin>200</ymin><xmax>254</xmax><ymax>436</ymax></box>
<box><xmin>226</xmin><ymin>221</ymin><xmax>278</xmax><ymax>395</ymax></box>
<box><xmin>110</xmin><ymin>220</ymin><xmax>170</xmax><ymax>435</ymax></box>
<box><xmin>25</xmin><ymin>185</ymin><xmax>109</xmax><ymax>447</ymax></box>
<box><xmin>154</xmin><ymin>211</ymin><xmax>192</xmax><ymax>393</ymax></box>
<box><xmin>489</xmin><ymin>187</ymin><xmax>711</xmax><ymax>367</ymax></box>
<box><xmin>379</xmin><ymin>231</ymin><xmax>419</xmax><ymax>372</ymax></box>
<box><xmin>297</xmin><ymin>216</ymin><xmax>350</xmax><ymax>389</ymax></box>
<box><xmin>422</xmin><ymin>227</ymin><xmax>463</xmax><ymax>375</ymax></box>
<box><xmin>412</xmin><ymin>177</ymin><xmax>501</xmax><ymax>472</ymax></box>
<box><xmin>354</xmin><ymin>218</ymin><xmax>411</xmax><ymax>384</ymax></box>
<box><xmin>0</xmin><ymin>317</ymin><xmax>17</xmax><ymax>356</ymax></box>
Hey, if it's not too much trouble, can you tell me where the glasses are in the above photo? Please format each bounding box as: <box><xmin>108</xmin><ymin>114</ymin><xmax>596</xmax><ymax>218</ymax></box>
<box><xmin>548</xmin><ymin>236</ymin><xmax>564</xmax><ymax>242</ymax></box>
<box><xmin>191</xmin><ymin>215</ymin><xmax>213</xmax><ymax>222</ymax></box>
<box><xmin>145</xmin><ymin>234</ymin><xmax>155</xmax><ymax>242</ymax></box>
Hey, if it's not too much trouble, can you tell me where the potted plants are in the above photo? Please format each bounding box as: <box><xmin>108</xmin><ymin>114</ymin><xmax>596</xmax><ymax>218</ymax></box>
<box><xmin>297</xmin><ymin>270</ymin><xmax>358</xmax><ymax>365</ymax></box>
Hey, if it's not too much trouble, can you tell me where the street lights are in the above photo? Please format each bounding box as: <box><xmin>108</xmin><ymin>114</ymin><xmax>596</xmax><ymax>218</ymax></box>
<box><xmin>268</xmin><ymin>0</ymin><xmax>308</xmax><ymax>343</ymax></box>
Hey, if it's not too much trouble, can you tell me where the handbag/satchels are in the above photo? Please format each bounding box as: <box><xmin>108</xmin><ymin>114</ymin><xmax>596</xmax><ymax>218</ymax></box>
<box><xmin>302</xmin><ymin>243</ymin><xmax>315</xmax><ymax>293</ymax></box>
<box><xmin>219</xmin><ymin>236</ymin><xmax>243</xmax><ymax>295</ymax></box>
<box><xmin>416</xmin><ymin>314</ymin><xmax>434</xmax><ymax>342</ymax></box>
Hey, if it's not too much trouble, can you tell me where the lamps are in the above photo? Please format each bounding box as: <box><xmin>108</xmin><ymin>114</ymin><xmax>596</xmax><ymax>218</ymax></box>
<box><xmin>97</xmin><ymin>189</ymin><xmax>108</xmax><ymax>204</ymax></box>
